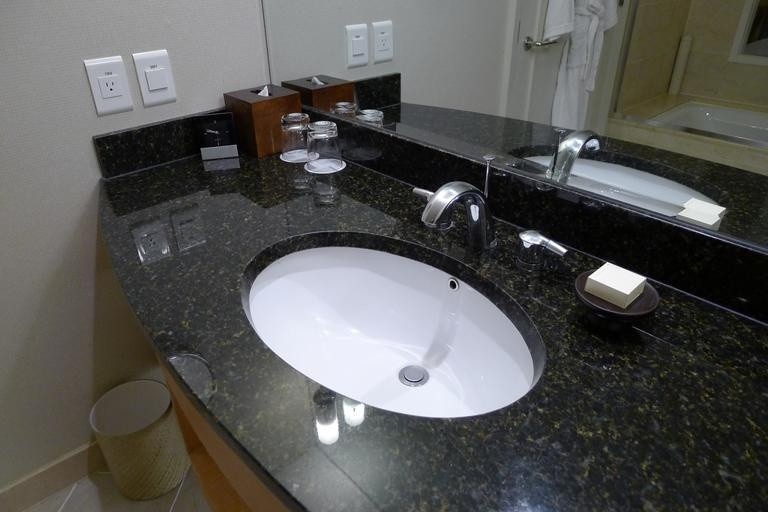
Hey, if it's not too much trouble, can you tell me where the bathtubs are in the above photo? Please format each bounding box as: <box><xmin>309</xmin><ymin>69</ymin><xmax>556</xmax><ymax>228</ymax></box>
<box><xmin>643</xmin><ymin>101</ymin><xmax>768</xmax><ymax>155</ymax></box>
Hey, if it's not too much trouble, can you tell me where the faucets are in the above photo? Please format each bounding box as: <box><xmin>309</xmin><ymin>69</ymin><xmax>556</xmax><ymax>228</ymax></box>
<box><xmin>544</xmin><ymin>127</ymin><xmax>601</xmax><ymax>185</ymax></box>
<box><xmin>413</xmin><ymin>181</ymin><xmax>497</xmax><ymax>251</ymax></box>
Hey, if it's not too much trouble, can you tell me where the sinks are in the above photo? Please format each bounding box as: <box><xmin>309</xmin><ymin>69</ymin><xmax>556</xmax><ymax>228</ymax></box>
<box><xmin>506</xmin><ymin>141</ymin><xmax>731</xmax><ymax>232</ymax></box>
<box><xmin>234</xmin><ymin>229</ymin><xmax>548</xmax><ymax>420</ymax></box>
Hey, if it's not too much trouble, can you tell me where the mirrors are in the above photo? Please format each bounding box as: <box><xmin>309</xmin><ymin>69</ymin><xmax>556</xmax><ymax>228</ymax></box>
<box><xmin>260</xmin><ymin>0</ymin><xmax>767</xmax><ymax>256</ymax></box>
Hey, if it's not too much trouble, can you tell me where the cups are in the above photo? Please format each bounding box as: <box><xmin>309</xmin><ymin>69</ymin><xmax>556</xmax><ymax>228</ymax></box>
<box><xmin>281</xmin><ymin>112</ymin><xmax>310</xmax><ymax>161</ymax></box>
<box><xmin>334</xmin><ymin>102</ymin><xmax>384</xmax><ymax>129</ymax></box>
<box><xmin>307</xmin><ymin>121</ymin><xmax>343</xmax><ymax>172</ymax></box>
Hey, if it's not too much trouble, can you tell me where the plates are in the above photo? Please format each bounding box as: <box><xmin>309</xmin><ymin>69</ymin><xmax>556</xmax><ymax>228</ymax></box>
<box><xmin>574</xmin><ymin>270</ymin><xmax>661</xmax><ymax>317</ymax></box>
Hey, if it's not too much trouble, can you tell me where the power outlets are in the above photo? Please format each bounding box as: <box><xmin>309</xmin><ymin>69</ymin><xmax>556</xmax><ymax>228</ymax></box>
<box><xmin>84</xmin><ymin>55</ymin><xmax>136</xmax><ymax>118</ymax></box>
<box><xmin>372</xmin><ymin>19</ymin><xmax>394</xmax><ymax>65</ymax></box>
